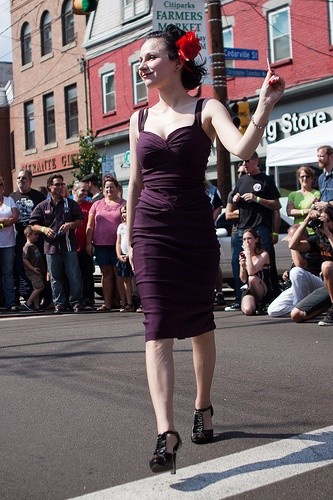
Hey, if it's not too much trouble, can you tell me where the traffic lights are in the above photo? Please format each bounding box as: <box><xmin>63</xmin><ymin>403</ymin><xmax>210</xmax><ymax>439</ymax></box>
<box><xmin>227</xmin><ymin>102</ymin><xmax>241</xmax><ymax>130</ymax></box>
<box><xmin>71</xmin><ymin>0</ymin><xmax>100</xmax><ymax>16</ymax></box>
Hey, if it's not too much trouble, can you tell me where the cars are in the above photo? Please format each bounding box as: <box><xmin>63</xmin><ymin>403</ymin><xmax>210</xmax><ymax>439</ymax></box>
<box><xmin>93</xmin><ymin>206</ymin><xmax>297</xmax><ymax>310</ymax></box>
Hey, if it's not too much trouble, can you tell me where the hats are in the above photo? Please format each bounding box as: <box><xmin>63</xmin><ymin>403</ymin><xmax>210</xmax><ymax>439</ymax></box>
<box><xmin>80</xmin><ymin>173</ymin><xmax>98</xmax><ymax>183</ymax></box>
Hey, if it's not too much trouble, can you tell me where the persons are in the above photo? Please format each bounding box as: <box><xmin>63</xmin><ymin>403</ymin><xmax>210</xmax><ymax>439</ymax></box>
<box><xmin>0</xmin><ymin>169</ymin><xmax>143</xmax><ymax>313</ymax></box>
<box><xmin>124</xmin><ymin>23</ymin><xmax>286</xmax><ymax>475</ymax></box>
<box><xmin>205</xmin><ymin>145</ymin><xmax>333</xmax><ymax>326</ymax></box>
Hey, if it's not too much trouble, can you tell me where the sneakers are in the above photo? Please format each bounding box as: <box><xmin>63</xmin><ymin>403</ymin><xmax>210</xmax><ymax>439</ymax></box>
<box><xmin>317</xmin><ymin>307</ymin><xmax>333</xmax><ymax>326</ymax></box>
<box><xmin>224</xmin><ymin>302</ymin><xmax>241</xmax><ymax>311</ymax></box>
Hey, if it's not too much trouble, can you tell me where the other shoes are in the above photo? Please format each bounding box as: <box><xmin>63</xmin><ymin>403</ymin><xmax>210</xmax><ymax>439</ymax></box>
<box><xmin>11</xmin><ymin>306</ymin><xmax>20</xmax><ymax>312</ymax></box>
<box><xmin>24</xmin><ymin>302</ymin><xmax>36</xmax><ymax>312</ymax></box>
<box><xmin>215</xmin><ymin>294</ymin><xmax>227</xmax><ymax>305</ymax></box>
<box><xmin>84</xmin><ymin>305</ymin><xmax>94</xmax><ymax>311</ymax></box>
<box><xmin>33</xmin><ymin>307</ymin><xmax>45</xmax><ymax>312</ymax></box>
<box><xmin>74</xmin><ymin>305</ymin><xmax>84</xmax><ymax>312</ymax></box>
<box><xmin>54</xmin><ymin>305</ymin><xmax>68</xmax><ymax>313</ymax></box>
<box><xmin>254</xmin><ymin>303</ymin><xmax>265</xmax><ymax>314</ymax></box>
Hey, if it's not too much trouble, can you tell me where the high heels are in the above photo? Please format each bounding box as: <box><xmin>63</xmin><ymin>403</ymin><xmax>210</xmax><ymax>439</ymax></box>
<box><xmin>191</xmin><ymin>402</ymin><xmax>213</xmax><ymax>444</ymax></box>
<box><xmin>149</xmin><ymin>430</ymin><xmax>182</xmax><ymax>474</ymax></box>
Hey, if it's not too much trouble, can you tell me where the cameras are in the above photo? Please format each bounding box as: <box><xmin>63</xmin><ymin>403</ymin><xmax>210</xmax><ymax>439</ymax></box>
<box><xmin>308</xmin><ymin>220</ymin><xmax>323</xmax><ymax>229</ymax></box>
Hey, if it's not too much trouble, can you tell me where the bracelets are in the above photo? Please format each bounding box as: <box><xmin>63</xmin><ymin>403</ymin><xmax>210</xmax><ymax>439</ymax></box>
<box><xmin>251</xmin><ymin>114</ymin><xmax>269</xmax><ymax>129</ymax></box>
<box><xmin>328</xmin><ymin>201</ymin><xmax>332</xmax><ymax>207</ymax></box>
<box><xmin>256</xmin><ymin>196</ymin><xmax>260</xmax><ymax>203</ymax></box>
<box><xmin>0</xmin><ymin>223</ymin><xmax>4</xmax><ymax>229</ymax></box>
<box><xmin>301</xmin><ymin>209</ymin><xmax>304</xmax><ymax>215</ymax></box>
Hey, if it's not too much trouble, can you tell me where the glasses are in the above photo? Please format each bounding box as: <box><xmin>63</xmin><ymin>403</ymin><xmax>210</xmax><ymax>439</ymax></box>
<box><xmin>17</xmin><ymin>176</ymin><xmax>31</xmax><ymax>180</ymax></box>
<box><xmin>236</xmin><ymin>171</ymin><xmax>246</xmax><ymax>175</ymax></box>
<box><xmin>50</xmin><ymin>182</ymin><xmax>65</xmax><ymax>187</ymax></box>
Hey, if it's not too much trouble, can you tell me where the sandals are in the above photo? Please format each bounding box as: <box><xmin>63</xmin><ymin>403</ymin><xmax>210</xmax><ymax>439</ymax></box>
<box><xmin>96</xmin><ymin>304</ymin><xmax>111</xmax><ymax>311</ymax></box>
<box><xmin>120</xmin><ymin>304</ymin><xmax>127</xmax><ymax>312</ymax></box>
<box><xmin>124</xmin><ymin>303</ymin><xmax>134</xmax><ymax>312</ymax></box>
<box><xmin>136</xmin><ymin>304</ymin><xmax>143</xmax><ymax>313</ymax></box>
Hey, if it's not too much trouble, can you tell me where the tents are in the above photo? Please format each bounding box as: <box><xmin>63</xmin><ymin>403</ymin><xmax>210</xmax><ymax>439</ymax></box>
<box><xmin>265</xmin><ymin>119</ymin><xmax>333</xmax><ymax>191</ymax></box>
